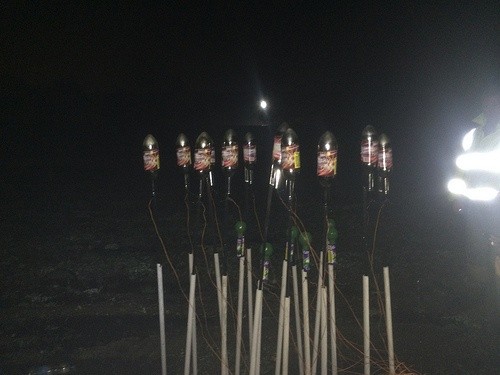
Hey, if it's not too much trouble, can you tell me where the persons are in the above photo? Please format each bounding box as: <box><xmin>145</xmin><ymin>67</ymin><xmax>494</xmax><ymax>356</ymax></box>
<box><xmin>450</xmin><ymin>81</ymin><xmax>499</xmax><ymax>331</ymax></box>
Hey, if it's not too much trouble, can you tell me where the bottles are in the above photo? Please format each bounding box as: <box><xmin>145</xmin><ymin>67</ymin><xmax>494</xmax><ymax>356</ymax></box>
<box><xmin>325</xmin><ymin>218</ymin><xmax>337</xmax><ymax>265</ymax></box>
<box><xmin>286</xmin><ymin>224</ymin><xmax>298</xmax><ymax>256</ymax></box>
<box><xmin>221</xmin><ymin>127</ymin><xmax>239</xmax><ymax>197</ymax></box>
<box><xmin>259</xmin><ymin>241</ymin><xmax>274</xmax><ymax>283</ymax></box>
<box><xmin>142</xmin><ymin>134</ymin><xmax>160</xmax><ymax>195</ymax></box>
<box><xmin>269</xmin><ymin>121</ymin><xmax>301</xmax><ymax>201</ymax></box>
<box><xmin>195</xmin><ymin>131</ymin><xmax>211</xmax><ymax>200</ymax></box>
<box><xmin>360</xmin><ymin>124</ymin><xmax>378</xmax><ymax>191</ymax></box>
<box><xmin>299</xmin><ymin>231</ymin><xmax>313</xmax><ymax>273</ymax></box>
<box><xmin>377</xmin><ymin>133</ymin><xmax>393</xmax><ymax>195</ymax></box>
<box><xmin>176</xmin><ymin>133</ymin><xmax>192</xmax><ymax>189</ymax></box>
<box><xmin>316</xmin><ymin>130</ymin><xmax>339</xmax><ymax>207</ymax></box>
<box><xmin>242</xmin><ymin>131</ymin><xmax>258</xmax><ymax>184</ymax></box>
<box><xmin>235</xmin><ymin>215</ymin><xmax>247</xmax><ymax>257</ymax></box>
<box><xmin>207</xmin><ymin>134</ymin><xmax>221</xmax><ymax>187</ymax></box>
